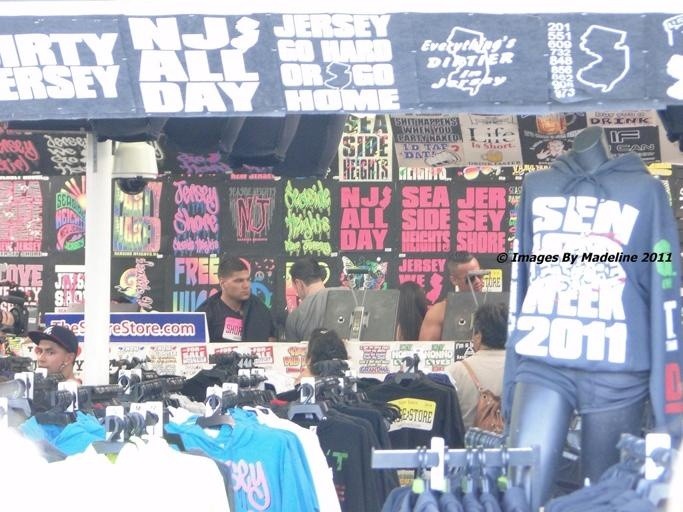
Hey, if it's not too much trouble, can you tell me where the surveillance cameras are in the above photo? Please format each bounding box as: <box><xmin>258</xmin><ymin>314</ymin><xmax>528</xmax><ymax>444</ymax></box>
<box><xmin>113</xmin><ymin>140</ymin><xmax>159</xmax><ymax>194</ymax></box>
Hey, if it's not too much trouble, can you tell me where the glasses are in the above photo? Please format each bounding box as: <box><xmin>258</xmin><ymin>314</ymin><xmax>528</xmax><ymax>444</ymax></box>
<box><xmin>465</xmin><ymin>273</ymin><xmax>484</xmax><ymax>283</ymax></box>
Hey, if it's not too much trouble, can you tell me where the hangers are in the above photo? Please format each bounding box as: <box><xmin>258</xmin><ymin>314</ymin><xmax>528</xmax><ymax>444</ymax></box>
<box><xmin>0</xmin><ymin>353</ymin><xmax>186</xmax><ymax>455</ymax></box>
<box><xmin>286</xmin><ymin>375</ymin><xmax>403</xmax><ymax>434</ymax></box>
<box><xmin>410</xmin><ymin>426</ymin><xmax>513</xmax><ymax>498</ymax></box>
<box><xmin>593</xmin><ymin>433</ymin><xmax>682</xmax><ymax>507</ymax></box>
<box><xmin>194</xmin><ymin>387</ymin><xmax>278</xmax><ymax>431</ymax></box>
<box><xmin>393</xmin><ymin>356</ymin><xmax>423</xmax><ymax>386</ymax></box>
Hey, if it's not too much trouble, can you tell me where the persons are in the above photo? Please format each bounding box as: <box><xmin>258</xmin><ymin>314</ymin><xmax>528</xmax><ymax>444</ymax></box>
<box><xmin>27</xmin><ymin>325</ymin><xmax>84</xmax><ymax>390</ymax></box>
<box><xmin>296</xmin><ymin>328</ymin><xmax>349</xmax><ymax>383</ymax></box>
<box><xmin>196</xmin><ymin>257</ymin><xmax>278</xmax><ymax>343</ymax></box>
<box><xmin>285</xmin><ymin>258</ymin><xmax>350</xmax><ymax>342</ymax></box>
<box><xmin>444</xmin><ymin>304</ymin><xmax>507</xmax><ymax>428</ymax></box>
<box><xmin>501</xmin><ymin>127</ymin><xmax>683</xmax><ymax>512</ymax></box>
<box><xmin>396</xmin><ymin>252</ymin><xmax>499</xmax><ymax>341</ymax></box>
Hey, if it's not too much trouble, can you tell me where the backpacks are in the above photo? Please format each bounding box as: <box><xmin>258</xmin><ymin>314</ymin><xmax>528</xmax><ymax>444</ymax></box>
<box><xmin>462</xmin><ymin>359</ymin><xmax>504</xmax><ymax>434</ymax></box>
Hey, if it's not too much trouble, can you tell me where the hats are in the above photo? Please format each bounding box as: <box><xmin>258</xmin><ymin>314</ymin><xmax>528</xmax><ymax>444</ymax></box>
<box><xmin>306</xmin><ymin>328</ymin><xmax>348</xmax><ymax>366</ymax></box>
<box><xmin>28</xmin><ymin>325</ymin><xmax>78</xmax><ymax>353</ymax></box>
